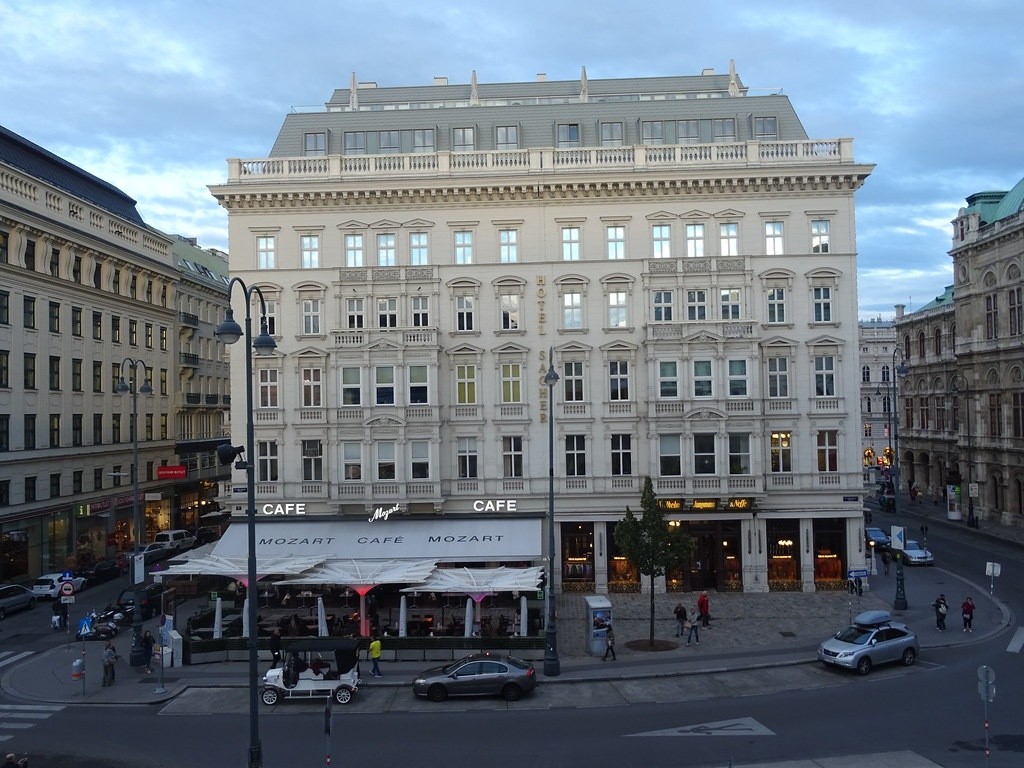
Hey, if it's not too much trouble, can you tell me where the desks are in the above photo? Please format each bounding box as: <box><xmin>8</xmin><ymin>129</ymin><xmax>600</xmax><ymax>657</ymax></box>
<box><xmin>339</xmin><ymin>592</ymin><xmax>354</xmax><ymax>608</ymax></box>
<box><xmin>487</xmin><ymin>593</ymin><xmax>499</xmax><ymax>608</ymax></box>
<box><xmin>195</xmin><ymin>614</ymin><xmax>479</xmax><ymax>633</ymax></box>
<box><xmin>441</xmin><ymin>593</ymin><xmax>467</xmax><ymax>608</ymax></box>
<box><xmin>296</xmin><ymin>594</ymin><xmax>324</xmax><ymax>609</ymax></box>
<box><xmin>507</xmin><ymin>614</ymin><xmax>521</xmax><ymax>634</ymax></box>
<box><xmin>260</xmin><ymin>593</ymin><xmax>274</xmax><ymax>608</ymax></box>
<box><xmin>407</xmin><ymin>593</ymin><xmax>422</xmax><ymax>608</ymax></box>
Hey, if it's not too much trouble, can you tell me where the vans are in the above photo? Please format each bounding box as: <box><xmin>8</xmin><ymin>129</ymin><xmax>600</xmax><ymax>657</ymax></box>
<box><xmin>0</xmin><ymin>583</ymin><xmax>36</xmax><ymax>619</ymax></box>
<box><xmin>154</xmin><ymin>529</ymin><xmax>197</xmax><ymax>553</ymax></box>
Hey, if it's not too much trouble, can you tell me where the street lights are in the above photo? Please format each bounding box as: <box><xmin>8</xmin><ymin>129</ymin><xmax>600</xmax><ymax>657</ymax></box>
<box><xmin>950</xmin><ymin>375</ymin><xmax>975</xmax><ymax>527</ymax></box>
<box><xmin>544</xmin><ymin>347</ymin><xmax>560</xmax><ymax>676</ymax></box>
<box><xmin>115</xmin><ymin>357</ymin><xmax>154</xmax><ymax>666</ymax></box>
<box><xmin>876</xmin><ymin>382</ymin><xmax>892</xmax><ymax>483</ymax></box>
<box><xmin>893</xmin><ymin>348</ymin><xmax>909</xmax><ymax>516</ymax></box>
<box><xmin>215</xmin><ymin>275</ymin><xmax>278</xmax><ymax>768</ymax></box>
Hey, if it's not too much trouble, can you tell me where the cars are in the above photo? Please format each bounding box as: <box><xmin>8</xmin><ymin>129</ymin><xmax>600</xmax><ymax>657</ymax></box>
<box><xmin>817</xmin><ymin>611</ymin><xmax>919</xmax><ymax>675</ymax></box>
<box><xmin>33</xmin><ymin>573</ymin><xmax>88</xmax><ymax>600</ymax></box>
<box><xmin>76</xmin><ymin>559</ymin><xmax>120</xmax><ymax>586</ymax></box>
<box><xmin>190</xmin><ymin>526</ymin><xmax>216</xmax><ymax>545</ymax></box>
<box><xmin>888</xmin><ymin>538</ymin><xmax>933</xmax><ymax>566</ymax></box>
<box><xmin>261</xmin><ymin>637</ymin><xmax>362</xmax><ymax>705</ymax></box>
<box><xmin>865</xmin><ymin>527</ymin><xmax>891</xmax><ymax>552</ymax></box>
<box><xmin>412</xmin><ymin>652</ymin><xmax>536</xmax><ymax>702</ymax></box>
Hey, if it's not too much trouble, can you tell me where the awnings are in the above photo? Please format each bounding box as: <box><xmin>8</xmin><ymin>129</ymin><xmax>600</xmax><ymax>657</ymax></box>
<box><xmin>211</xmin><ymin>518</ymin><xmax>543</xmax><ymax>561</ymax></box>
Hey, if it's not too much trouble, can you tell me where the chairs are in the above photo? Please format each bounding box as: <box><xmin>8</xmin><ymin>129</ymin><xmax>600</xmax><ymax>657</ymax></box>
<box><xmin>227</xmin><ymin>614</ymin><xmax>514</xmax><ymax>637</ymax></box>
<box><xmin>299</xmin><ymin>663</ymin><xmax>309</xmax><ymax>679</ymax></box>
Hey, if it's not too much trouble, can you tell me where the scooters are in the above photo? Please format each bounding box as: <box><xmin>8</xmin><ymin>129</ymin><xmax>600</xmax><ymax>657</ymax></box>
<box><xmin>76</xmin><ymin>601</ymin><xmax>135</xmax><ymax>640</ymax></box>
<box><xmin>113</xmin><ymin>552</ymin><xmax>128</xmax><ymax>574</ymax></box>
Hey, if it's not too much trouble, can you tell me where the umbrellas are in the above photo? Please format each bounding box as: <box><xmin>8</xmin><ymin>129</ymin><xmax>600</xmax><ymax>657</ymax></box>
<box><xmin>727</xmin><ymin>58</ymin><xmax>740</xmax><ymax>97</ymax></box>
<box><xmin>580</xmin><ymin>66</ymin><xmax>588</xmax><ymax>103</ymax></box>
<box><xmin>470</xmin><ymin>69</ymin><xmax>479</xmax><ymax>106</ymax></box>
<box><xmin>350</xmin><ymin>72</ymin><xmax>358</xmax><ymax>110</ymax></box>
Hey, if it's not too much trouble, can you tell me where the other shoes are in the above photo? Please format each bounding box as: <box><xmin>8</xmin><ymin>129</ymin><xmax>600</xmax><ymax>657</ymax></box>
<box><xmin>600</xmin><ymin>657</ymin><xmax>606</xmax><ymax>662</ymax></box>
<box><xmin>369</xmin><ymin>670</ymin><xmax>374</xmax><ymax>675</ymax></box>
<box><xmin>611</xmin><ymin>658</ymin><xmax>615</xmax><ymax>660</ymax></box>
<box><xmin>375</xmin><ymin>674</ymin><xmax>382</xmax><ymax>678</ymax></box>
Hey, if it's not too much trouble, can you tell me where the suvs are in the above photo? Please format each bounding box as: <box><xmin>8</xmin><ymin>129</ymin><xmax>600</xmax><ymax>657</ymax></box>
<box><xmin>117</xmin><ymin>582</ymin><xmax>173</xmax><ymax>619</ymax></box>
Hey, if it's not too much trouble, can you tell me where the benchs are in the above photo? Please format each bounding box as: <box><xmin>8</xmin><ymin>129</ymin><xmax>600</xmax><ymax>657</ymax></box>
<box><xmin>337</xmin><ymin>654</ymin><xmax>358</xmax><ymax>680</ymax></box>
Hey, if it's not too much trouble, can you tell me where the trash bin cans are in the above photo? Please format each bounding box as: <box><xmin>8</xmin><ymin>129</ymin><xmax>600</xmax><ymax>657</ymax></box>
<box><xmin>71</xmin><ymin>659</ymin><xmax>83</xmax><ymax>681</ymax></box>
<box><xmin>155</xmin><ymin>647</ymin><xmax>171</xmax><ymax>667</ymax></box>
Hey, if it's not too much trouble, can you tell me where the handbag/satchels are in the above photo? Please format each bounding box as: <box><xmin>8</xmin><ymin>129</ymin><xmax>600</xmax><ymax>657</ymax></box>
<box><xmin>684</xmin><ymin>619</ymin><xmax>692</xmax><ymax>630</ymax></box>
<box><xmin>103</xmin><ymin>655</ymin><xmax>117</xmax><ymax>667</ymax></box>
<box><xmin>606</xmin><ymin>636</ymin><xmax>614</xmax><ymax>647</ymax></box>
<box><xmin>882</xmin><ymin>553</ymin><xmax>887</xmax><ymax>563</ymax></box>
<box><xmin>963</xmin><ymin>613</ymin><xmax>971</xmax><ymax>620</ymax></box>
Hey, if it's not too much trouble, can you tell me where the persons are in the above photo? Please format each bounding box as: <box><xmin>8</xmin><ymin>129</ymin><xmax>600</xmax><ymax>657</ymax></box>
<box><xmin>908</xmin><ymin>479</ymin><xmax>948</xmax><ymax>505</ymax></box>
<box><xmin>102</xmin><ymin>641</ymin><xmax>119</xmax><ymax>687</ymax></box>
<box><xmin>960</xmin><ymin>597</ymin><xmax>976</xmax><ymax>632</ymax></box>
<box><xmin>366</xmin><ymin>635</ymin><xmax>383</xmax><ymax>677</ymax></box>
<box><xmin>269</xmin><ymin>628</ymin><xmax>305</xmax><ymax>688</ymax></box>
<box><xmin>881</xmin><ymin>549</ymin><xmax>892</xmax><ymax>576</ymax></box>
<box><xmin>2</xmin><ymin>754</ymin><xmax>20</xmax><ymax>768</ymax></box>
<box><xmin>931</xmin><ymin>594</ymin><xmax>949</xmax><ymax>631</ymax></box>
<box><xmin>600</xmin><ymin>625</ymin><xmax>617</xmax><ymax>662</ymax></box>
<box><xmin>674</xmin><ymin>590</ymin><xmax>712</xmax><ymax>646</ymax></box>
<box><xmin>141</xmin><ymin>630</ymin><xmax>156</xmax><ymax>673</ymax></box>
